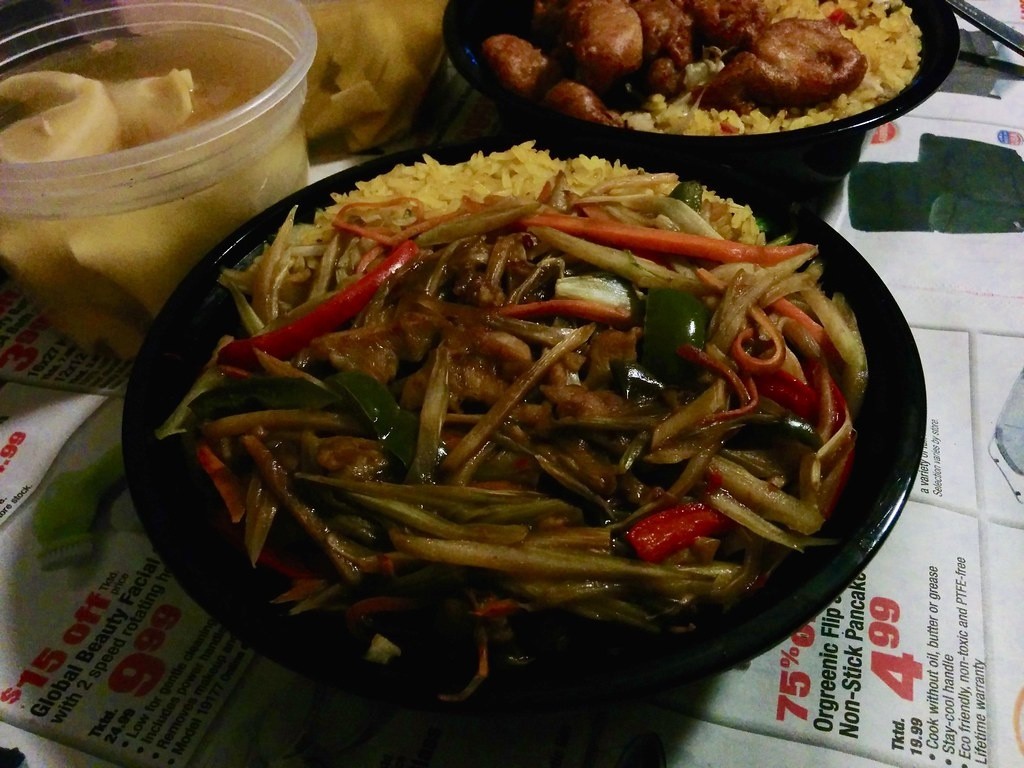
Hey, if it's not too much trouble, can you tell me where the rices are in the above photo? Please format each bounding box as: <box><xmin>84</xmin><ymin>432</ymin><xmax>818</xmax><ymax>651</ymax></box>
<box><xmin>483</xmin><ymin>0</ymin><xmax>923</xmax><ymax>137</ymax></box>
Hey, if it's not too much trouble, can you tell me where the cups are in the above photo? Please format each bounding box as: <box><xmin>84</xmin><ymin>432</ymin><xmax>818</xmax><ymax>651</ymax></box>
<box><xmin>4</xmin><ymin>0</ymin><xmax>324</xmax><ymax>363</ymax></box>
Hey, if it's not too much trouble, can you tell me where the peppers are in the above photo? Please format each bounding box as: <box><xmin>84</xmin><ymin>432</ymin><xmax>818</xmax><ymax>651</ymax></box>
<box><xmin>156</xmin><ymin>140</ymin><xmax>872</xmax><ymax>651</ymax></box>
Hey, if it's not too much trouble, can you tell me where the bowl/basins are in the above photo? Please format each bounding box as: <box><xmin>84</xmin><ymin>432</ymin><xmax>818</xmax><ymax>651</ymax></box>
<box><xmin>123</xmin><ymin>142</ymin><xmax>931</xmax><ymax>708</ymax></box>
<box><xmin>439</xmin><ymin>2</ymin><xmax>962</xmax><ymax>221</ymax></box>
